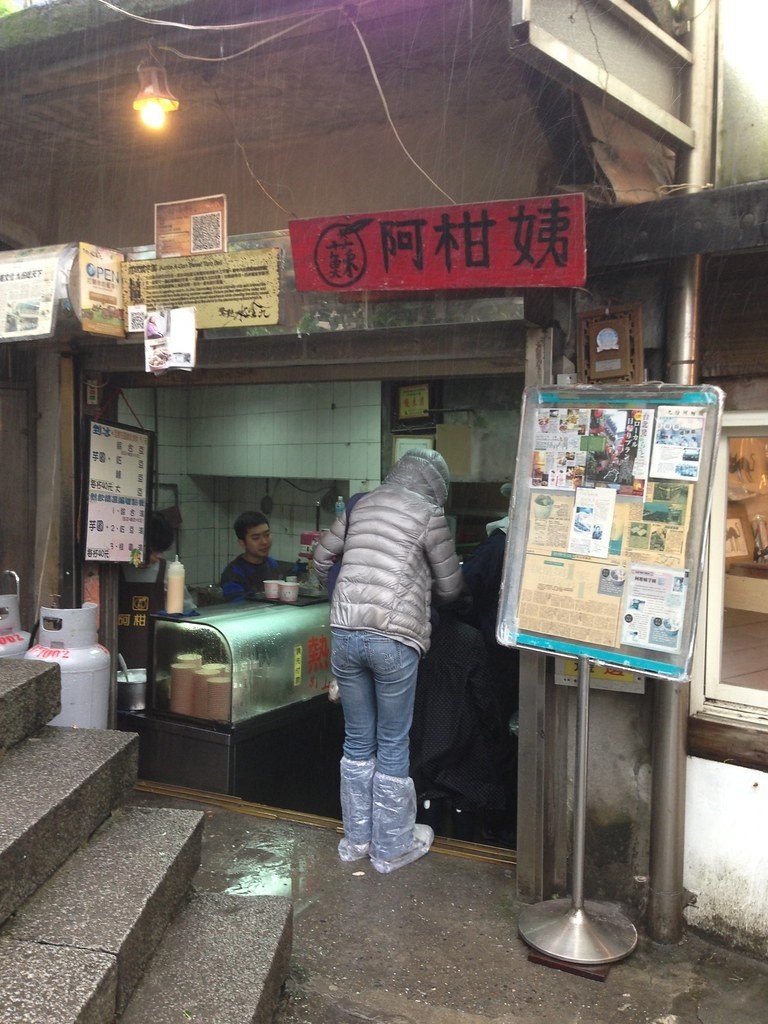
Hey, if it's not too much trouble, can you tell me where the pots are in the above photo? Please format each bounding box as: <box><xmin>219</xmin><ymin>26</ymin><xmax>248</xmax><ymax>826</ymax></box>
<box><xmin>247</xmin><ymin>666</ymin><xmax>295</xmax><ymax>705</ymax></box>
<box><xmin>117</xmin><ymin>668</ymin><xmax>168</xmax><ymax>710</ymax></box>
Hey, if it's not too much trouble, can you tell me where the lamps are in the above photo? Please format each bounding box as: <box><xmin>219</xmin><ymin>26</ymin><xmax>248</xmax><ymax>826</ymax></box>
<box><xmin>133</xmin><ymin>49</ymin><xmax>178</xmax><ymax>130</ymax></box>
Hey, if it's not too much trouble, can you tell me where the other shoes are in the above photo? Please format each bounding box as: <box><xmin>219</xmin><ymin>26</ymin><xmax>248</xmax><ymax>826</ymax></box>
<box><xmin>370</xmin><ymin>824</ymin><xmax>435</xmax><ymax>873</ymax></box>
<box><xmin>338</xmin><ymin>835</ymin><xmax>369</xmax><ymax>862</ymax></box>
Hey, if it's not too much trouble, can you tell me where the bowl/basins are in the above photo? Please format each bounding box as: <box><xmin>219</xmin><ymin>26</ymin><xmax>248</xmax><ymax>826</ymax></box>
<box><xmin>278</xmin><ymin>582</ymin><xmax>300</xmax><ymax>602</ymax></box>
<box><xmin>170</xmin><ymin>653</ymin><xmax>232</xmax><ymax>721</ymax></box>
<box><xmin>263</xmin><ymin>580</ymin><xmax>285</xmax><ymax>599</ymax></box>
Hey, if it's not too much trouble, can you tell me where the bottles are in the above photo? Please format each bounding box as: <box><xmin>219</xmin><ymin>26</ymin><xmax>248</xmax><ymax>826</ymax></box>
<box><xmin>335</xmin><ymin>496</ymin><xmax>345</xmax><ymax>520</ymax></box>
<box><xmin>165</xmin><ymin>555</ymin><xmax>185</xmax><ymax>614</ymax></box>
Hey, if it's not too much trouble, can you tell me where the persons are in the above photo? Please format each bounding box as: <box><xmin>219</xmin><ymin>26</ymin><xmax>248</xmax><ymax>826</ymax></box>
<box><xmin>456</xmin><ymin>483</ymin><xmax>518</xmax><ymax>838</ymax></box>
<box><xmin>592</xmin><ymin>526</ymin><xmax>602</xmax><ymax>539</ymax></box>
<box><xmin>312</xmin><ymin>446</ymin><xmax>466</xmax><ymax>877</ymax></box>
<box><xmin>115</xmin><ymin>511</ymin><xmax>197</xmax><ymax>674</ymax></box>
<box><xmin>408</xmin><ymin>583</ymin><xmax>506</xmax><ymax>843</ymax></box>
<box><xmin>219</xmin><ymin>510</ymin><xmax>308</xmax><ymax>605</ymax></box>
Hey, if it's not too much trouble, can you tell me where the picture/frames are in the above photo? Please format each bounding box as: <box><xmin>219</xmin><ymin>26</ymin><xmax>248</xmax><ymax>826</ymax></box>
<box><xmin>726</xmin><ymin>502</ymin><xmax>756</xmax><ymax>566</ymax></box>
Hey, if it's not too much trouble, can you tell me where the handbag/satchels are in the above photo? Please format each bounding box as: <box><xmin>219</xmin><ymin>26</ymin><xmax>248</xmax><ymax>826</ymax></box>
<box><xmin>327</xmin><ymin>493</ymin><xmax>367</xmax><ymax>603</ymax></box>
